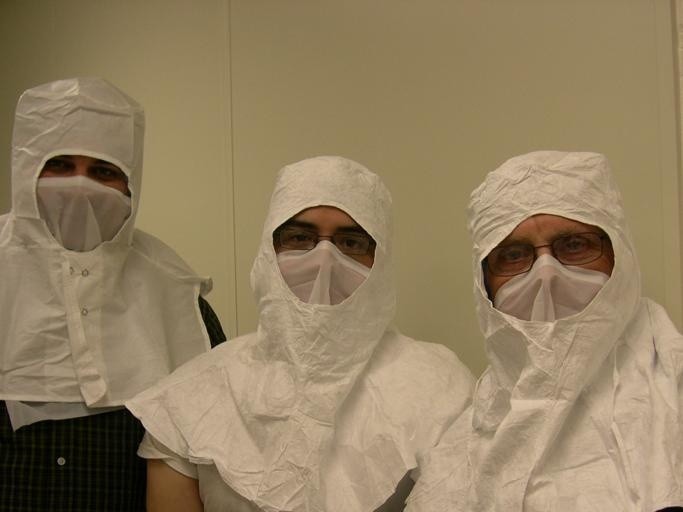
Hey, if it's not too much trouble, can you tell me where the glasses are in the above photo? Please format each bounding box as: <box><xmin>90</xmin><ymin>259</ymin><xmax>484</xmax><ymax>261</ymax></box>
<box><xmin>485</xmin><ymin>232</ymin><xmax>605</xmax><ymax>277</ymax></box>
<box><xmin>279</xmin><ymin>228</ymin><xmax>373</xmax><ymax>256</ymax></box>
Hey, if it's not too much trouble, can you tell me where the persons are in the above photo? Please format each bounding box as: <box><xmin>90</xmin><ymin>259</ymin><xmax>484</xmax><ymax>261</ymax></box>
<box><xmin>123</xmin><ymin>155</ymin><xmax>476</xmax><ymax>511</ymax></box>
<box><xmin>401</xmin><ymin>151</ymin><xmax>683</xmax><ymax>512</ymax></box>
<box><xmin>0</xmin><ymin>79</ymin><xmax>228</xmax><ymax>512</ymax></box>
